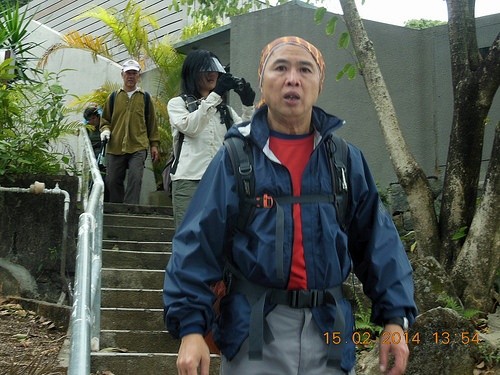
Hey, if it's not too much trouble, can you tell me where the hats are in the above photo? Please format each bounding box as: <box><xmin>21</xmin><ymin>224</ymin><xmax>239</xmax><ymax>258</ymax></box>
<box><xmin>199</xmin><ymin>57</ymin><xmax>226</xmax><ymax>73</ymax></box>
<box><xmin>121</xmin><ymin>59</ymin><xmax>140</xmax><ymax>72</ymax></box>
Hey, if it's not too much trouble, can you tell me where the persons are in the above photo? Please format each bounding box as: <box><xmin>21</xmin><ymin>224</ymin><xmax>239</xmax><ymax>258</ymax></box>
<box><xmin>168</xmin><ymin>50</ymin><xmax>256</xmax><ymax>232</ymax></box>
<box><xmin>100</xmin><ymin>61</ymin><xmax>160</xmax><ymax>205</ymax></box>
<box><xmin>163</xmin><ymin>37</ymin><xmax>418</xmax><ymax>375</ymax></box>
<box><xmin>84</xmin><ymin>105</ymin><xmax>102</xmax><ymax>160</ymax></box>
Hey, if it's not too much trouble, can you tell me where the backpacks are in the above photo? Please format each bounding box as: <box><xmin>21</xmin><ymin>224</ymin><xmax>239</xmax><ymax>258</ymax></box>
<box><xmin>162</xmin><ymin>95</ymin><xmax>198</xmax><ymax>198</ymax></box>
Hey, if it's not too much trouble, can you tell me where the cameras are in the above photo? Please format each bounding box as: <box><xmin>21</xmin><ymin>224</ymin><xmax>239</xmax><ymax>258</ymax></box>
<box><xmin>228</xmin><ymin>76</ymin><xmax>244</xmax><ymax>90</ymax></box>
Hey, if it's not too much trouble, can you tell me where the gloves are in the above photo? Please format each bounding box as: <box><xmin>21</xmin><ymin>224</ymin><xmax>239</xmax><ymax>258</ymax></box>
<box><xmin>213</xmin><ymin>73</ymin><xmax>235</xmax><ymax>97</ymax></box>
<box><xmin>100</xmin><ymin>130</ymin><xmax>110</xmax><ymax>142</ymax></box>
<box><xmin>234</xmin><ymin>78</ymin><xmax>256</xmax><ymax>106</ymax></box>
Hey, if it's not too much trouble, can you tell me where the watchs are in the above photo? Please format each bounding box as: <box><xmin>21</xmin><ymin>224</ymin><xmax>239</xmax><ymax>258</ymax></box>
<box><xmin>383</xmin><ymin>317</ymin><xmax>409</xmax><ymax>331</ymax></box>
